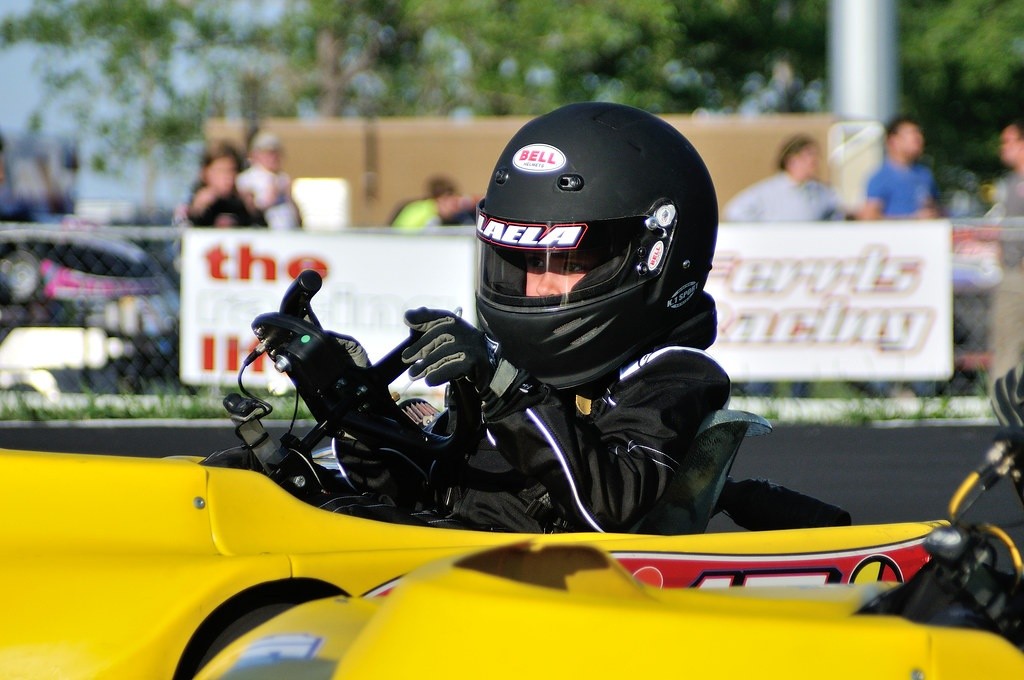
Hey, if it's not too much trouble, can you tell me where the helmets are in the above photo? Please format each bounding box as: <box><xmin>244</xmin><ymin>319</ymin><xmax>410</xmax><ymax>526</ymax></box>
<box><xmin>475</xmin><ymin>101</ymin><xmax>717</xmax><ymax>392</ymax></box>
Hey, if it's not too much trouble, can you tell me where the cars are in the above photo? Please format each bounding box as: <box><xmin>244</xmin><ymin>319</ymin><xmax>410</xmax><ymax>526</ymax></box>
<box><xmin>0</xmin><ymin>221</ymin><xmax>182</xmax><ymax>386</ymax></box>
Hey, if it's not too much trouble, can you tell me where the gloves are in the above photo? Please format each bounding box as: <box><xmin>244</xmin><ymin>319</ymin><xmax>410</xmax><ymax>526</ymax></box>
<box><xmin>399</xmin><ymin>307</ymin><xmax>527</xmax><ymax>418</ymax></box>
<box><xmin>321</xmin><ymin>330</ymin><xmax>373</xmax><ymax>368</ymax></box>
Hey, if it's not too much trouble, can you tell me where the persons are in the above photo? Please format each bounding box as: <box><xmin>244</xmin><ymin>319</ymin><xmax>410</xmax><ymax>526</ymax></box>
<box><xmin>317</xmin><ymin>100</ymin><xmax>731</xmax><ymax>535</ymax></box>
<box><xmin>856</xmin><ymin>119</ymin><xmax>942</xmax><ymax>399</ymax></box>
<box><xmin>725</xmin><ymin>133</ymin><xmax>849</xmax><ymax>395</ymax></box>
<box><xmin>187</xmin><ymin>130</ymin><xmax>301</xmax><ymax>227</ymax></box>
<box><xmin>388</xmin><ymin>176</ymin><xmax>482</xmax><ymax>230</ymax></box>
<box><xmin>990</xmin><ymin>123</ymin><xmax>1024</xmax><ymax>392</ymax></box>
